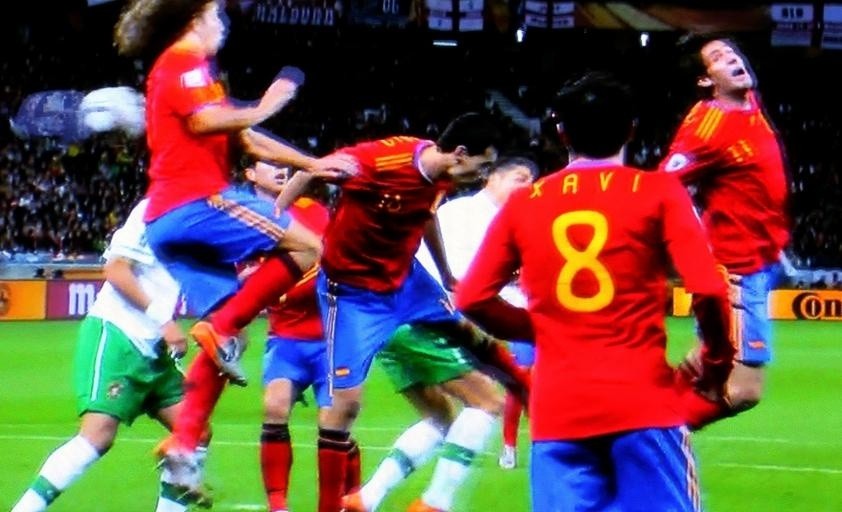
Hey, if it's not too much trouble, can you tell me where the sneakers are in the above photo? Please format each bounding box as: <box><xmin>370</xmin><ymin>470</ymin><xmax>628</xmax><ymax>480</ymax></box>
<box><xmin>188</xmin><ymin>322</ymin><xmax>249</xmax><ymax>387</ymax></box>
<box><xmin>155</xmin><ymin>438</ymin><xmax>212</xmax><ymax>507</ymax></box>
<box><xmin>499</xmin><ymin>445</ymin><xmax>515</xmax><ymax>468</ymax></box>
<box><xmin>340</xmin><ymin>491</ymin><xmax>442</xmax><ymax>511</ymax></box>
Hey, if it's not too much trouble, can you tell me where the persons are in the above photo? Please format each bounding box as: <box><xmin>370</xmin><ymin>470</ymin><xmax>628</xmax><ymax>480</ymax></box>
<box><xmin>217</xmin><ymin>66</ymin><xmax>702</xmax><ymax>214</ymax></box>
<box><xmin>500</xmin><ymin>326</ymin><xmax>534</xmax><ymax>473</ymax></box>
<box><xmin>112</xmin><ymin>0</ymin><xmax>360</xmax><ymax>512</ymax></box>
<box><xmin>358</xmin><ymin>156</ymin><xmax>539</xmax><ymax>510</ymax></box>
<box><xmin>1</xmin><ymin>71</ymin><xmax>152</xmax><ymax>275</ymax></box>
<box><xmin>756</xmin><ymin>72</ymin><xmax>842</xmax><ymax>275</ymax></box>
<box><xmin>645</xmin><ymin>35</ymin><xmax>794</xmax><ymax>434</ymax></box>
<box><xmin>272</xmin><ymin>111</ymin><xmax>533</xmax><ymax>512</ymax></box>
<box><xmin>243</xmin><ymin>143</ymin><xmax>362</xmax><ymax>512</ymax></box>
<box><xmin>457</xmin><ymin>72</ymin><xmax>740</xmax><ymax>511</ymax></box>
<box><xmin>11</xmin><ymin>138</ymin><xmax>326</xmax><ymax>511</ymax></box>
